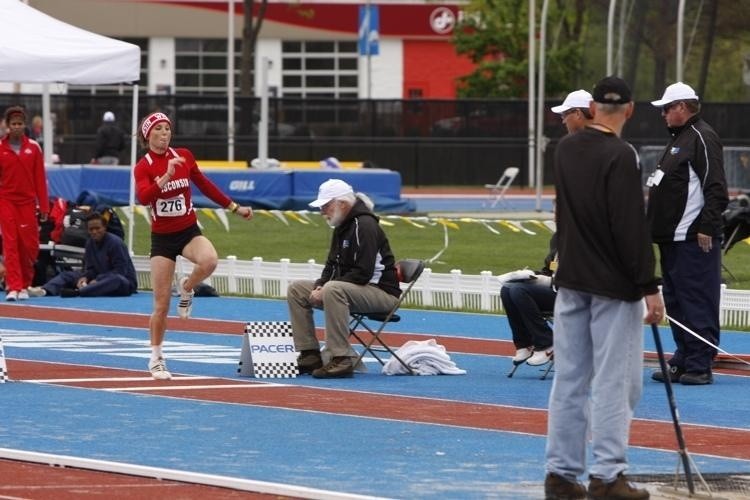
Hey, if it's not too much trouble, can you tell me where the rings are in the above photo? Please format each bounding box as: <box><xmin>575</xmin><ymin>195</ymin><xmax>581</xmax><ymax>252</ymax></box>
<box><xmin>654</xmin><ymin>310</ymin><xmax>660</xmax><ymax>316</ymax></box>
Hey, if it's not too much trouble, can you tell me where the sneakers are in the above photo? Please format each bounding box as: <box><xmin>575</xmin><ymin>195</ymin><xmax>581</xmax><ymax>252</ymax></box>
<box><xmin>680</xmin><ymin>369</ymin><xmax>713</xmax><ymax>384</ymax></box>
<box><xmin>296</xmin><ymin>354</ymin><xmax>322</xmax><ymax>374</ymax></box>
<box><xmin>148</xmin><ymin>358</ymin><xmax>172</xmax><ymax>379</ymax></box>
<box><xmin>5</xmin><ymin>290</ymin><xmax>18</xmax><ymax>300</ymax></box>
<box><xmin>28</xmin><ymin>286</ymin><xmax>46</xmax><ymax>297</ymax></box>
<box><xmin>589</xmin><ymin>473</ymin><xmax>649</xmax><ymax>500</ymax></box>
<box><xmin>513</xmin><ymin>345</ymin><xmax>535</xmax><ymax>365</ymax></box>
<box><xmin>545</xmin><ymin>473</ymin><xmax>588</xmax><ymax>500</ymax></box>
<box><xmin>177</xmin><ymin>277</ymin><xmax>195</xmax><ymax>317</ymax></box>
<box><xmin>18</xmin><ymin>288</ymin><xmax>29</xmax><ymax>300</ymax></box>
<box><xmin>526</xmin><ymin>346</ymin><xmax>553</xmax><ymax>366</ymax></box>
<box><xmin>652</xmin><ymin>365</ymin><xmax>683</xmax><ymax>382</ymax></box>
<box><xmin>313</xmin><ymin>360</ymin><xmax>352</xmax><ymax>377</ymax></box>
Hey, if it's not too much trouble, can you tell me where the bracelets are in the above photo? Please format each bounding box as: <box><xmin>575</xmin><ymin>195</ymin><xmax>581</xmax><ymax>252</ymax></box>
<box><xmin>231</xmin><ymin>203</ymin><xmax>240</xmax><ymax>214</ymax></box>
<box><xmin>166</xmin><ymin>172</ymin><xmax>172</xmax><ymax>177</ymax></box>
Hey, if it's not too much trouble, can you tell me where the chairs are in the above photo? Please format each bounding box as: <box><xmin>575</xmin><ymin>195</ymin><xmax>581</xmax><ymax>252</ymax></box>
<box><xmin>720</xmin><ymin>222</ymin><xmax>744</xmax><ymax>284</ymax></box>
<box><xmin>337</xmin><ymin>258</ymin><xmax>426</xmax><ymax>376</ymax></box>
<box><xmin>484</xmin><ymin>167</ymin><xmax>520</xmax><ymax>210</ymax></box>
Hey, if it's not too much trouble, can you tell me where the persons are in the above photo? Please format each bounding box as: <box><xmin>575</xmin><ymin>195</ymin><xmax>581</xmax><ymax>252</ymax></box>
<box><xmin>286</xmin><ymin>177</ymin><xmax>401</xmax><ymax>380</ymax></box>
<box><xmin>501</xmin><ymin>197</ymin><xmax>560</xmax><ymax>367</ymax></box>
<box><xmin>133</xmin><ymin>109</ymin><xmax>256</xmax><ymax>380</ymax></box>
<box><xmin>544</xmin><ymin>75</ymin><xmax>665</xmax><ymax>499</ymax></box>
<box><xmin>0</xmin><ymin>98</ymin><xmax>138</xmax><ymax>301</ymax></box>
<box><xmin>646</xmin><ymin>81</ymin><xmax>729</xmax><ymax>388</ymax></box>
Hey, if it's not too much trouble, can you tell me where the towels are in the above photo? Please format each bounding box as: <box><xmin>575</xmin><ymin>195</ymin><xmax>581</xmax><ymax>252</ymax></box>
<box><xmin>381</xmin><ymin>338</ymin><xmax>467</xmax><ymax>377</ymax></box>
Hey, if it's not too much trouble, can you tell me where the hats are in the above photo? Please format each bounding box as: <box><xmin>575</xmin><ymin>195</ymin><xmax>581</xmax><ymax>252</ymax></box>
<box><xmin>551</xmin><ymin>89</ymin><xmax>594</xmax><ymax>114</ymax></box>
<box><xmin>651</xmin><ymin>82</ymin><xmax>699</xmax><ymax>107</ymax></box>
<box><xmin>308</xmin><ymin>179</ymin><xmax>352</xmax><ymax>208</ymax></box>
<box><xmin>593</xmin><ymin>76</ymin><xmax>630</xmax><ymax>104</ymax></box>
